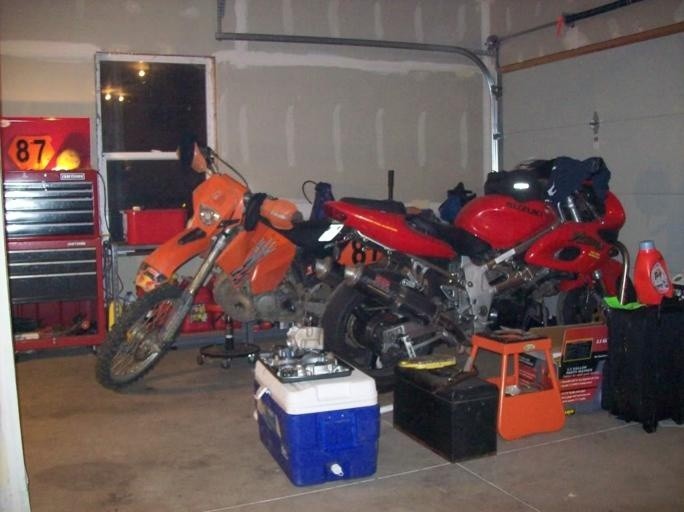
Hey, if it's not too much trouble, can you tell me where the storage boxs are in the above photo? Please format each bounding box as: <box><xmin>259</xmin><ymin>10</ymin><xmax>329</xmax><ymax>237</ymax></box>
<box><xmin>253</xmin><ymin>352</ymin><xmax>498</xmax><ymax>488</ymax></box>
<box><xmin>178</xmin><ymin>285</ymin><xmax>242</xmax><ymax>331</ymax></box>
<box><xmin>120</xmin><ymin>207</ymin><xmax>188</xmax><ymax>246</ymax></box>
<box><xmin>493</xmin><ymin>322</ymin><xmax>608</xmax><ymax>404</ymax></box>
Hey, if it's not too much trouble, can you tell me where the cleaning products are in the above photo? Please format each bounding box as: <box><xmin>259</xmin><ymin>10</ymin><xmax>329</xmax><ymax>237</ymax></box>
<box><xmin>633</xmin><ymin>239</ymin><xmax>675</xmax><ymax>305</ymax></box>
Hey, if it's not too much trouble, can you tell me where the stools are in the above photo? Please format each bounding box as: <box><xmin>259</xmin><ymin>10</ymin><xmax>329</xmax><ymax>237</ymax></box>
<box><xmin>464</xmin><ymin>329</ymin><xmax>566</xmax><ymax>438</ymax></box>
